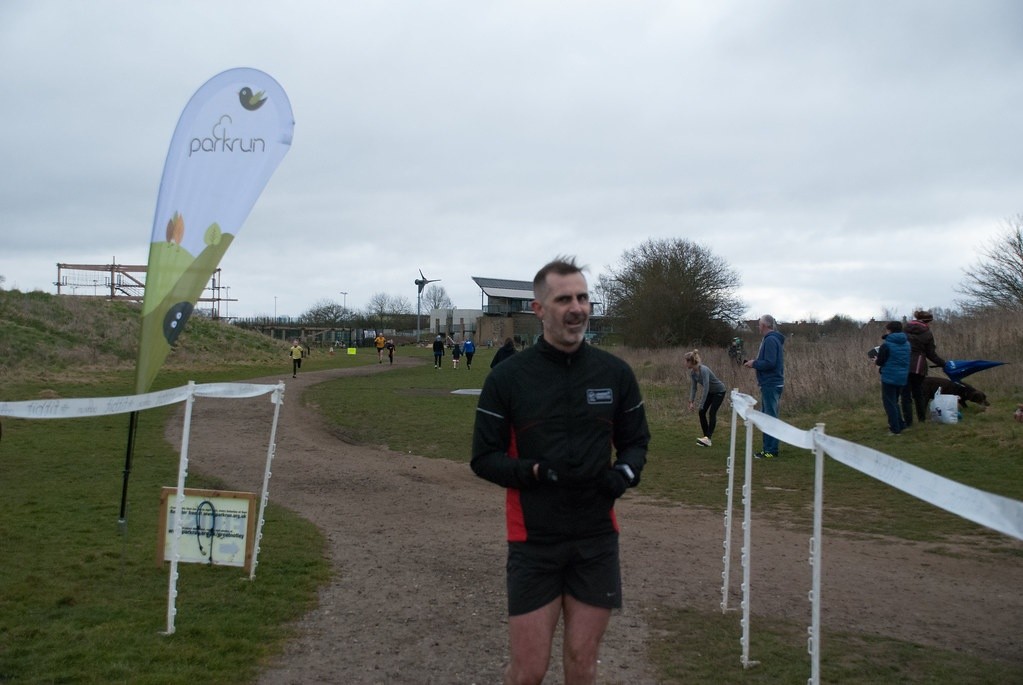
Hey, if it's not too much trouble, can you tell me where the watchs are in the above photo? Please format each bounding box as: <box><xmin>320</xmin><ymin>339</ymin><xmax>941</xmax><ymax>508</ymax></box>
<box><xmin>614</xmin><ymin>464</ymin><xmax>635</xmax><ymax>483</ymax></box>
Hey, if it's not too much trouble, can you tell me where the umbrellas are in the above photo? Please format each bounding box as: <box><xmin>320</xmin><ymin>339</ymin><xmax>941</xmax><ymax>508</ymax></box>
<box><xmin>928</xmin><ymin>359</ymin><xmax>1010</xmax><ymax>382</ymax></box>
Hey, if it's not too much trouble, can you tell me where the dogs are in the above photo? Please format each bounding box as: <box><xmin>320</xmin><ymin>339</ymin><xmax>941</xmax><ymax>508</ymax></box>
<box><xmin>919</xmin><ymin>377</ymin><xmax>990</xmax><ymax>409</ymax></box>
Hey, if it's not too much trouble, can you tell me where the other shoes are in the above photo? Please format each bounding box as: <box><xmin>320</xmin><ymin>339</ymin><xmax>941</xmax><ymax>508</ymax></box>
<box><xmin>439</xmin><ymin>367</ymin><xmax>443</xmax><ymax>370</ymax></box>
<box><xmin>380</xmin><ymin>359</ymin><xmax>382</xmax><ymax>364</ymax></box>
<box><xmin>468</xmin><ymin>365</ymin><xmax>471</xmax><ymax>370</ymax></box>
<box><xmin>293</xmin><ymin>375</ymin><xmax>297</xmax><ymax>378</ymax></box>
<box><xmin>435</xmin><ymin>365</ymin><xmax>437</xmax><ymax>368</ymax></box>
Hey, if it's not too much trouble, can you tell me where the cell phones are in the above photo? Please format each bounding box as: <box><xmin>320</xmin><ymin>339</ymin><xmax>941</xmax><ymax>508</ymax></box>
<box><xmin>743</xmin><ymin>360</ymin><xmax>747</xmax><ymax>364</ymax></box>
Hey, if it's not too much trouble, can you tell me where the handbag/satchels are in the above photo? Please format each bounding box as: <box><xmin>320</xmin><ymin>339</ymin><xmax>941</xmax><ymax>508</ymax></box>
<box><xmin>928</xmin><ymin>387</ymin><xmax>961</xmax><ymax>425</ymax></box>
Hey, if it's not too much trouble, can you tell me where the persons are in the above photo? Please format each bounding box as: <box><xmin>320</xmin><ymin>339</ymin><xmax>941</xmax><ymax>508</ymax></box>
<box><xmin>903</xmin><ymin>310</ymin><xmax>946</xmax><ymax>426</ymax></box>
<box><xmin>489</xmin><ymin>338</ymin><xmax>520</xmax><ymax>372</ymax></box>
<box><xmin>289</xmin><ymin>339</ymin><xmax>304</xmax><ymax>378</ymax></box>
<box><xmin>352</xmin><ymin>339</ymin><xmax>358</xmax><ymax>349</ymax></box>
<box><xmin>384</xmin><ymin>338</ymin><xmax>396</xmax><ymax>364</ymax></box>
<box><xmin>871</xmin><ymin>321</ymin><xmax>912</xmax><ymax>435</ymax></box>
<box><xmin>451</xmin><ymin>344</ymin><xmax>463</xmax><ymax>369</ymax></box>
<box><xmin>432</xmin><ymin>334</ymin><xmax>445</xmax><ymax>369</ymax></box>
<box><xmin>374</xmin><ymin>333</ymin><xmax>386</xmax><ymax>364</ymax></box>
<box><xmin>462</xmin><ymin>337</ymin><xmax>476</xmax><ymax>370</ymax></box>
<box><xmin>684</xmin><ymin>349</ymin><xmax>727</xmax><ymax>447</ymax></box>
<box><xmin>336</xmin><ymin>339</ymin><xmax>344</xmax><ymax>347</ymax></box>
<box><xmin>743</xmin><ymin>314</ymin><xmax>785</xmax><ymax>459</ymax></box>
<box><xmin>469</xmin><ymin>257</ymin><xmax>652</xmax><ymax>685</ymax></box>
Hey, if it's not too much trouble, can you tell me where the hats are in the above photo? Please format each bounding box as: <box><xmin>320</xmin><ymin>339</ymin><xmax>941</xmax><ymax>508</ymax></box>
<box><xmin>886</xmin><ymin>321</ymin><xmax>902</xmax><ymax>333</ymax></box>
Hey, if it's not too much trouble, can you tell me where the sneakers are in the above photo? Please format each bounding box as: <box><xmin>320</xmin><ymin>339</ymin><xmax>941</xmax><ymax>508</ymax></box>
<box><xmin>696</xmin><ymin>436</ymin><xmax>713</xmax><ymax>447</ymax></box>
<box><xmin>754</xmin><ymin>451</ymin><xmax>779</xmax><ymax>460</ymax></box>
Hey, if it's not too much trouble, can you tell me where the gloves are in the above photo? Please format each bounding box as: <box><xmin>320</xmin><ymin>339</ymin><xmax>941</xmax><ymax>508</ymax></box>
<box><xmin>533</xmin><ymin>462</ymin><xmax>574</xmax><ymax>507</ymax></box>
<box><xmin>573</xmin><ymin>467</ymin><xmax>627</xmax><ymax>507</ymax></box>
